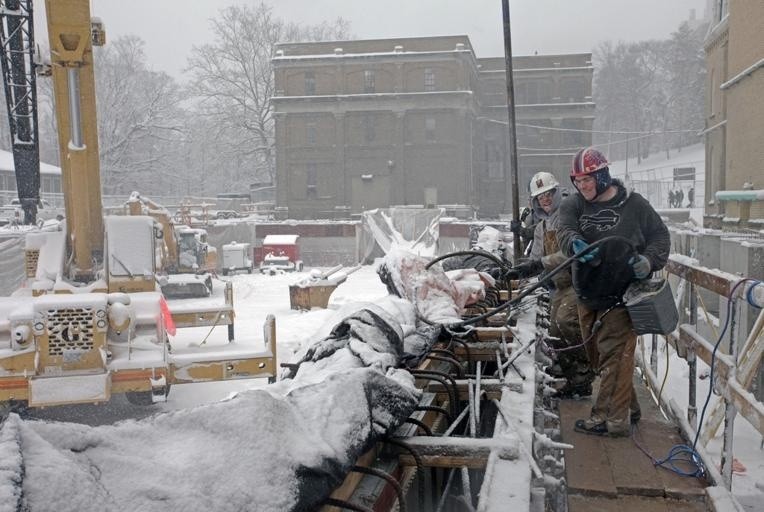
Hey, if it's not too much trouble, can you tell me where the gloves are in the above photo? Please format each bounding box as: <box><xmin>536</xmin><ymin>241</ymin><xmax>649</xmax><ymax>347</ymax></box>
<box><xmin>512</xmin><ymin>257</ymin><xmax>542</xmax><ymax>278</ymax></box>
<box><xmin>572</xmin><ymin>237</ymin><xmax>600</xmax><ymax>264</ymax></box>
<box><xmin>629</xmin><ymin>254</ymin><xmax>652</xmax><ymax>280</ymax></box>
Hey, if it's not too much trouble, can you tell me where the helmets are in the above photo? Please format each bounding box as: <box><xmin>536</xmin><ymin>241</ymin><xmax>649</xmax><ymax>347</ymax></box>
<box><xmin>529</xmin><ymin>171</ymin><xmax>560</xmax><ymax>197</ymax></box>
<box><xmin>569</xmin><ymin>148</ymin><xmax>609</xmax><ymax>177</ymax></box>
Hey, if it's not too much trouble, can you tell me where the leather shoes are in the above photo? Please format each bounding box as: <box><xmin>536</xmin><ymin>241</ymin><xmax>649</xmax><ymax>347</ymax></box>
<box><xmin>551</xmin><ymin>379</ymin><xmax>593</xmax><ymax>400</ymax></box>
<box><xmin>575</xmin><ymin>408</ymin><xmax>643</xmax><ymax>438</ymax></box>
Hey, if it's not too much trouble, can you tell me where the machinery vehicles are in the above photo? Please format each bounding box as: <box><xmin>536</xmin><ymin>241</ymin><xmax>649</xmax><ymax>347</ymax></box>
<box><xmin>0</xmin><ymin>0</ymin><xmax>277</xmax><ymax>422</ymax></box>
<box><xmin>125</xmin><ymin>189</ymin><xmax>218</xmax><ymax>299</ymax></box>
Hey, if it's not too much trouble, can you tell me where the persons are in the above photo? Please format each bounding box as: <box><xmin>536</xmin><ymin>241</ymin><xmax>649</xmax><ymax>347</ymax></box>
<box><xmin>558</xmin><ymin>148</ymin><xmax>672</xmax><ymax>436</ymax></box>
<box><xmin>510</xmin><ymin>181</ymin><xmax>569</xmax><ymax>377</ymax></box>
<box><xmin>500</xmin><ymin>172</ymin><xmax>641</xmax><ymax>423</ymax></box>
<box><xmin>669</xmin><ymin>186</ymin><xmax>695</xmax><ymax>209</ymax></box>
